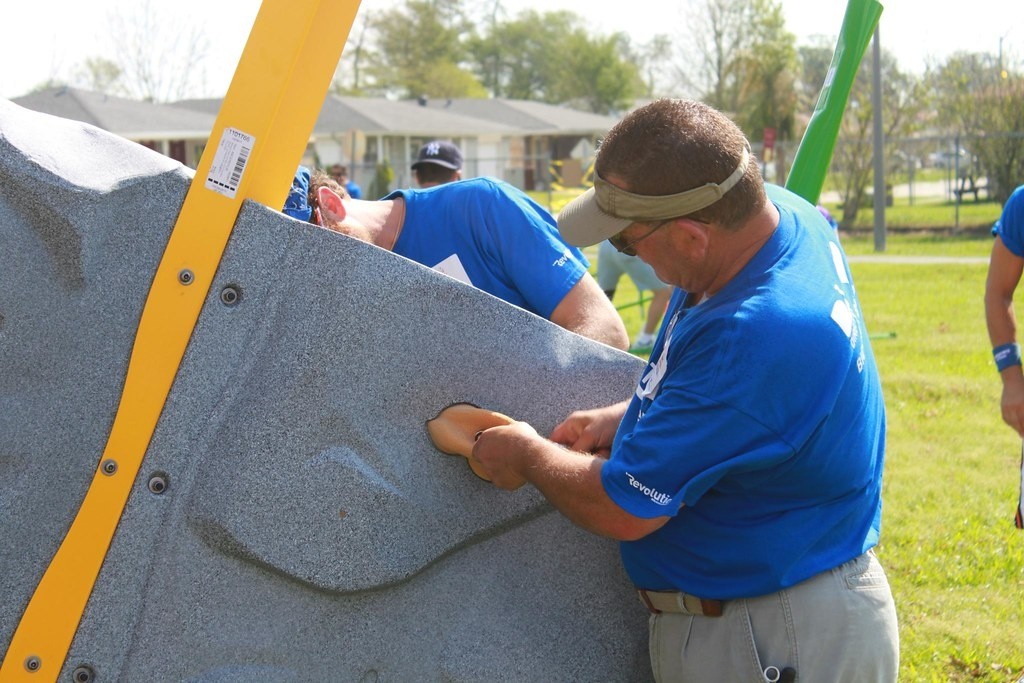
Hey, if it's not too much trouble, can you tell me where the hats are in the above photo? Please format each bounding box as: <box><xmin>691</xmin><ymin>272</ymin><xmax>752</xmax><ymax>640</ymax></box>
<box><xmin>557</xmin><ymin>137</ymin><xmax>751</xmax><ymax>247</ymax></box>
<box><xmin>410</xmin><ymin>140</ymin><xmax>463</xmax><ymax>170</ymax></box>
<box><xmin>283</xmin><ymin>164</ymin><xmax>312</xmax><ymax>222</ymax></box>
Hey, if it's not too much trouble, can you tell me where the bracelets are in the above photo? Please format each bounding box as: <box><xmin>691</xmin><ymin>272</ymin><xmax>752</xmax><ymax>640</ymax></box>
<box><xmin>993</xmin><ymin>342</ymin><xmax>1022</xmax><ymax>372</ymax></box>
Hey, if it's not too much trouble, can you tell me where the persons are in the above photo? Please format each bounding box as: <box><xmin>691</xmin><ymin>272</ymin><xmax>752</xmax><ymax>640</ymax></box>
<box><xmin>282</xmin><ymin>166</ymin><xmax>633</xmax><ymax>352</ymax></box>
<box><xmin>331</xmin><ymin>164</ymin><xmax>362</xmax><ymax>199</ymax></box>
<box><xmin>409</xmin><ymin>140</ymin><xmax>464</xmax><ymax>189</ymax></box>
<box><xmin>473</xmin><ymin>99</ymin><xmax>900</xmax><ymax>683</ymax></box>
<box><xmin>596</xmin><ymin>239</ymin><xmax>673</xmax><ymax>348</ymax></box>
<box><xmin>983</xmin><ymin>186</ymin><xmax>1024</xmax><ymax>530</ymax></box>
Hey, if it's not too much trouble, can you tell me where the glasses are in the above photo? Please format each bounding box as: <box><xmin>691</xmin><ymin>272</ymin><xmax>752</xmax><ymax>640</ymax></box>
<box><xmin>417</xmin><ymin>164</ymin><xmax>456</xmax><ymax>185</ymax></box>
<box><xmin>608</xmin><ymin>216</ymin><xmax>710</xmax><ymax>256</ymax></box>
<box><xmin>333</xmin><ymin>173</ymin><xmax>344</xmax><ymax>177</ymax></box>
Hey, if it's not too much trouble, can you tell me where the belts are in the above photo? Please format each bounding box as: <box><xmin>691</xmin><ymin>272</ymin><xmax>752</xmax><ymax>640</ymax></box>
<box><xmin>639</xmin><ymin>589</ymin><xmax>723</xmax><ymax>617</ymax></box>
